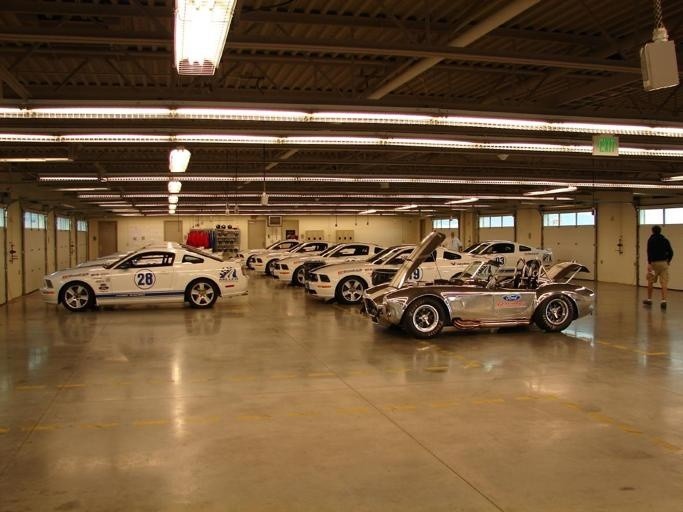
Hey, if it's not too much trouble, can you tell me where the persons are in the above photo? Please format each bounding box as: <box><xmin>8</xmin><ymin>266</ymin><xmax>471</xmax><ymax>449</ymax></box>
<box><xmin>440</xmin><ymin>231</ymin><xmax>462</xmax><ymax>252</ymax></box>
<box><xmin>641</xmin><ymin>224</ymin><xmax>674</xmax><ymax>308</ymax></box>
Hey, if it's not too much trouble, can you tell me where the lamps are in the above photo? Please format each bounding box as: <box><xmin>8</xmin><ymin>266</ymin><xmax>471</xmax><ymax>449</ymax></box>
<box><xmin>0</xmin><ymin>98</ymin><xmax>683</xmax><ymax>217</ymax></box>
<box><xmin>171</xmin><ymin>0</ymin><xmax>238</xmax><ymax>76</ymax></box>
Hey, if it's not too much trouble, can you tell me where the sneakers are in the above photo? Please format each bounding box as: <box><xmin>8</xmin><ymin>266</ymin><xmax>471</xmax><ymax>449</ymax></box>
<box><xmin>642</xmin><ymin>300</ymin><xmax>653</xmax><ymax>306</ymax></box>
<box><xmin>660</xmin><ymin>301</ymin><xmax>668</xmax><ymax>310</ymax></box>
